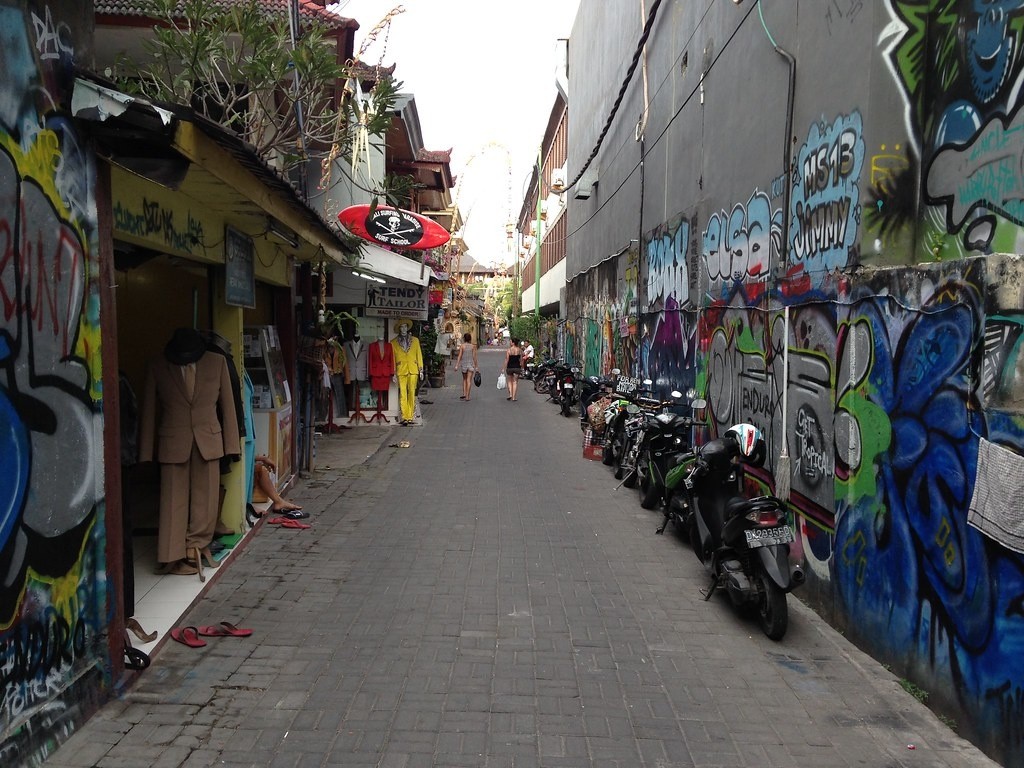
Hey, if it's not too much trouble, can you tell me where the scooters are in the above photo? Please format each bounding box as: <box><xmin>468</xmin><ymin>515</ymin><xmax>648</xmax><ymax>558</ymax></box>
<box><xmin>526</xmin><ymin>351</ymin><xmax>710</xmax><ymax>536</ymax></box>
<box><xmin>674</xmin><ymin>436</ymin><xmax>808</xmax><ymax>642</ymax></box>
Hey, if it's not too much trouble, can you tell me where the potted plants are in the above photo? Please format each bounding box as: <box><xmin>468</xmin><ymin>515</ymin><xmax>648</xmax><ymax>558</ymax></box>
<box><xmin>434</xmin><ymin>355</ymin><xmax>446</xmax><ymax>385</ymax></box>
<box><xmin>431</xmin><ymin>369</ymin><xmax>443</xmax><ymax>387</ymax></box>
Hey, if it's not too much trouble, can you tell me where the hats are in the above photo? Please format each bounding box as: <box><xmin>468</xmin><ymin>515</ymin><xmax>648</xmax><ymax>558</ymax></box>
<box><xmin>394</xmin><ymin>318</ymin><xmax>413</xmax><ymax>333</ymax></box>
<box><xmin>165</xmin><ymin>327</ymin><xmax>206</xmax><ymax>365</ymax></box>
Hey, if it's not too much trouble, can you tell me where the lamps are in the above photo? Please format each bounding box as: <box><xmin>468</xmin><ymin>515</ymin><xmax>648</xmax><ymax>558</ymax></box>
<box><xmin>266</xmin><ymin>216</ymin><xmax>300</xmax><ymax>249</ymax></box>
<box><xmin>352</xmin><ymin>269</ymin><xmax>387</xmax><ymax>283</ymax></box>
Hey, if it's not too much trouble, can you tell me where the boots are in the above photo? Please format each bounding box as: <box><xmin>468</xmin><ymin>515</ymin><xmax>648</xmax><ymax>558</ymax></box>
<box><xmin>215</xmin><ymin>485</ymin><xmax>236</xmax><ymax>536</ymax></box>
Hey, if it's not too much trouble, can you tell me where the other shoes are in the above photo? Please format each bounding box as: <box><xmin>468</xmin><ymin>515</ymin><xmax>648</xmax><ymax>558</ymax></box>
<box><xmin>162</xmin><ymin>561</ymin><xmax>197</xmax><ymax>576</ymax></box>
<box><xmin>460</xmin><ymin>396</ymin><xmax>465</xmax><ymax>398</ymax></box>
<box><xmin>403</xmin><ymin>419</ymin><xmax>408</xmax><ymax>426</ymax></box>
<box><xmin>408</xmin><ymin>420</ymin><xmax>415</xmax><ymax>424</ymax></box>
<box><xmin>507</xmin><ymin>397</ymin><xmax>511</xmax><ymax>400</ymax></box>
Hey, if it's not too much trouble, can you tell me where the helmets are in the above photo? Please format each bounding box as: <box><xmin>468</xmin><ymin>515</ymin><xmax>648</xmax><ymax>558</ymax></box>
<box><xmin>723</xmin><ymin>423</ymin><xmax>766</xmax><ymax>468</ymax></box>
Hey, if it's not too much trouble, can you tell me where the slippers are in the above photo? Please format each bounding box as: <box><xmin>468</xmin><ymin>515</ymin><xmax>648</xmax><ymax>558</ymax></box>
<box><xmin>268</xmin><ymin>516</ymin><xmax>291</xmax><ymax>523</ymax></box>
<box><xmin>420</xmin><ymin>400</ymin><xmax>433</xmax><ymax>404</ymax></box>
<box><xmin>282</xmin><ymin>520</ymin><xmax>311</xmax><ymax>529</ymax></box>
<box><xmin>171</xmin><ymin>626</ymin><xmax>207</xmax><ymax>647</ymax></box>
<box><xmin>273</xmin><ymin>508</ymin><xmax>295</xmax><ymax>513</ymax></box>
<box><xmin>284</xmin><ymin>509</ymin><xmax>309</xmax><ymax>519</ymax></box>
<box><xmin>197</xmin><ymin>621</ymin><xmax>253</xmax><ymax>637</ymax></box>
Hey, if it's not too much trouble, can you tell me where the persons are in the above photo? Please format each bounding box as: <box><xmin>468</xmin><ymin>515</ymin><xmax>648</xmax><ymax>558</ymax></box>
<box><xmin>494</xmin><ymin>330</ymin><xmax>503</xmax><ymax>346</ymax></box>
<box><xmin>502</xmin><ymin>337</ymin><xmax>507</xmax><ymax>345</ymax></box>
<box><xmin>500</xmin><ymin>336</ymin><xmax>524</xmax><ymax>401</ymax></box>
<box><xmin>322</xmin><ymin>336</ymin><xmax>350</xmax><ymax>418</ymax></box>
<box><xmin>521</xmin><ymin>340</ymin><xmax>534</xmax><ymax>376</ymax></box>
<box><xmin>390</xmin><ymin>319</ymin><xmax>423</xmax><ymax>426</ymax></box>
<box><xmin>244</xmin><ymin>367</ymin><xmax>256</xmax><ymax>519</ymax></box>
<box><xmin>487</xmin><ymin>337</ymin><xmax>493</xmax><ymax>346</ymax></box>
<box><xmin>342</xmin><ymin>333</ymin><xmax>369</xmax><ymax>383</ymax></box>
<box><xmin>141</xmin><ymin>330</ymin><xmax>241</xmax><ymax>576</ymax></box>
<box><xmin>252</xmin><ymin>454</ymin><xmax>304</xmax><ymax>512</ymax></box>
<box><xmin>454</xmin><ymin>333</ymin><xmax>479</xmax><ymax>401</ymax></box>
<box><xmin>492</xmin><ymin>336</ymin><xmax>500</xmax><ymax>346</ymax></box>
<box><xmin>201</xmin><ymin>331</ymin><xmax>247</xmax><ymax>558</ymax></box>
<box><xmin>368</xmin><ymin>337</ymin><xmax>394</xmax><ymax>392</ymax></box>
<box><xmin>484</xmin><ymin>332</ymin><xmax>491</xmax><ymax>341</ymax></box>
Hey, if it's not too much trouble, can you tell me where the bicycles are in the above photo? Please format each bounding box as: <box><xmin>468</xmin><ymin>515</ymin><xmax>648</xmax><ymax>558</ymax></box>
<box><xmin>497</xmin><ymin>339</ymin><xmax>507</xmax><ymax>345</ymax></box>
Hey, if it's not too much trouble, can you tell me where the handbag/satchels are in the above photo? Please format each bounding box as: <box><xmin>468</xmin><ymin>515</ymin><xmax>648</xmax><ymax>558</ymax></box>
<box><xmin>296</xmin><ymin>335</ymin><xmax>327</xmax><ymax>365</ymax></box>
<box><xmin>474</xmin><ymin>372</ymin><xmax>482</xmax><ymax>387</ymax></box>
<box><xmin>497</xmin><ymin>373</ymin><xmax>507</xmax><ymax>389</ymax></box>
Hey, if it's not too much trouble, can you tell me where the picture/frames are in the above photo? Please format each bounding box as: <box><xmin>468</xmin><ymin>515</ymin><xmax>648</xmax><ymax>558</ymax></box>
<box><xmin>224</xmin><ymin>224</ymin><xmax>257</xmax><ymax>309</ymax></box>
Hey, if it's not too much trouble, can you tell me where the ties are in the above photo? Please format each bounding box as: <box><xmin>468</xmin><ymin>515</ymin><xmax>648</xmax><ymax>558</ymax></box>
<box><xmin>184</xmin><ymin>362</ymin><xmax>196</xmax><ymax>400</ymax></box>
<box><xmin>403</xmin><ymin>337</ymin><xmax>408</xmax><ymax>351</ymax></box>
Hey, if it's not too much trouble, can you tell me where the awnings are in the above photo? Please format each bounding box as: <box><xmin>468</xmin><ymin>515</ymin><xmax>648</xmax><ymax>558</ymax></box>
<box><xmin>352</xmin><ymin>237</ymin><xmax>440</xmax><ymax>287</ymax></box>
